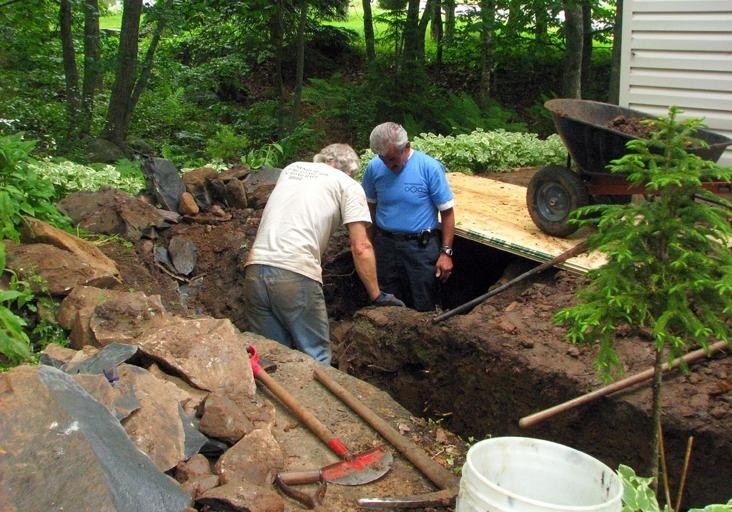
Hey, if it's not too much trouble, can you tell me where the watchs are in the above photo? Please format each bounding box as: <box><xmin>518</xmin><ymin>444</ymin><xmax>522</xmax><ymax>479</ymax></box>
<box><xmin>437</xmin><ymin>245</ymin><xmax>454</xmax><ymax>257</ymax></box>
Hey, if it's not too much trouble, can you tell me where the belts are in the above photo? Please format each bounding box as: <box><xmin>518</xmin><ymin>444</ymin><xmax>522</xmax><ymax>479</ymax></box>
<box><xmin>374</xmin><ymin>225</ymin><xmax>439</xmax><ymax>242</ymax></box>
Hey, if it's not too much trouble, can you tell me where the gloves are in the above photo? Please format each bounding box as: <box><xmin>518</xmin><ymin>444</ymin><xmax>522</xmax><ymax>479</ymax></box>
<box><xmin>371</xmin><ymin>290</ymin><xmax>406</xmax><ymax>308</ymax></box>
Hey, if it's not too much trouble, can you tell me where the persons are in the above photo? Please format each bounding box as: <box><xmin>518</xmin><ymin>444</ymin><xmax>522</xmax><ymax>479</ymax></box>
<box><xmin>360</xmin><ymin>120</ymin><xmax>459</xmax><ymax>312</ymax></box>
<box><xmin>241</xmin><ymin>141</ymin><xmax>408</xmax><ymax>368</ymax></box>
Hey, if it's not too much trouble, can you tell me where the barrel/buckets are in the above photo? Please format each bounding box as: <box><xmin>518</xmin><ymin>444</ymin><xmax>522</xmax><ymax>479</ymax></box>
<box><xmin>454</xmin><ymin>437</ymin><xmax>624</xmax><ymax>511</ymax></box>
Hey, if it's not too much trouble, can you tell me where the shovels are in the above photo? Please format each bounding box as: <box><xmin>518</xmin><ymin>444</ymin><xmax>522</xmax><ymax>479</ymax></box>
<box><xmin>242</xmin><ymin>341</ymin><xmax>394</xmax><ymax>487</ymax></box>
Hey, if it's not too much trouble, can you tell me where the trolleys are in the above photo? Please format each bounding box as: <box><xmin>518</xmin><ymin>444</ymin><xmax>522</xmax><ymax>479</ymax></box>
<box><xmin>524</xmin><ymin>93</ymin><xmax>732</xmax><ymax>239</ymax></box>
